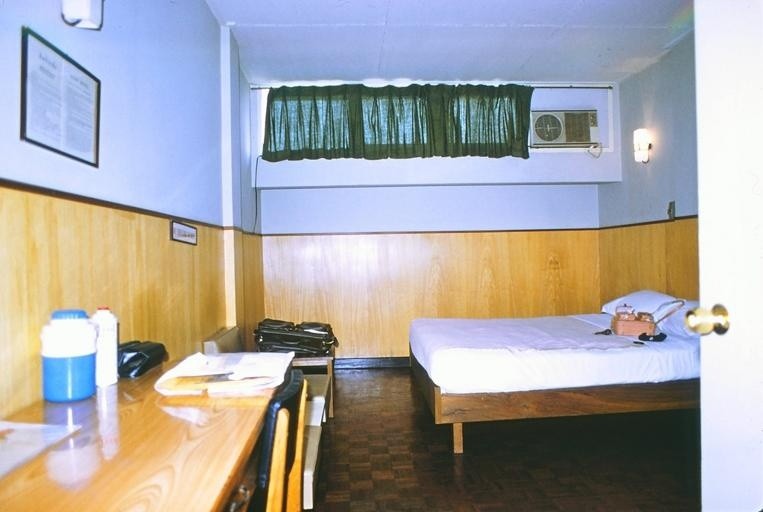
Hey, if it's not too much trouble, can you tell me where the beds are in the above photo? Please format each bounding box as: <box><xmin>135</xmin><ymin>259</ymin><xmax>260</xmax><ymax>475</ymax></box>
<box><xmin>409</xmin><ymin>314</ymin><xmax>699</xmax><ymax>453</ymax></box>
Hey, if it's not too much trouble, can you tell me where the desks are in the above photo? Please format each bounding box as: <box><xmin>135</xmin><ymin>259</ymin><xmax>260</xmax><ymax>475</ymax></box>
<box><xmin>1</xmin><ymin>352</ymin><xmax>303</xmax><ymax>511</ymax></box>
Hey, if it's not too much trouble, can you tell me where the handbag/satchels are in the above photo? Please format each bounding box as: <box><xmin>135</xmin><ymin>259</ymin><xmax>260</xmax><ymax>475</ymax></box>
<box><xmin>610</xmin><ymin>300</ymin><xmax>685</xmax><ymax>337</ymax></box>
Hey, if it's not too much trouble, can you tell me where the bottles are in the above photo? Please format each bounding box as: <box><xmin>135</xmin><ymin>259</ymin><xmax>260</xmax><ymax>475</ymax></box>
<box><xmin>92</xmin><ymin>305</ymin><xmax>119</xmax><ymax>386</ymax></box>
<box><xmin>41</xmin><ymin>310</ymin><xmax>98</xmax><ymax>403</ymax></box>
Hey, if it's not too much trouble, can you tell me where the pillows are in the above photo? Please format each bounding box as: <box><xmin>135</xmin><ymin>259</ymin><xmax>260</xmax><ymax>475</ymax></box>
<box><xmin>601</xmin><ymin>289</ymin><xmax>698</xmax><ymax>342</ymax></box>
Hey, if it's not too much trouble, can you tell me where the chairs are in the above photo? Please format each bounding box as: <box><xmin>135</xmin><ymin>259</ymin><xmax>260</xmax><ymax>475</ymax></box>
<box><xmin>249</xmin><ymin>365</ymin><xmax>303</xmax><ymax>510</ymax></box>
<box><xmin>195</xmin><ymin>326</ymin><xmax>335</xmax><ymax>422</ymax></box>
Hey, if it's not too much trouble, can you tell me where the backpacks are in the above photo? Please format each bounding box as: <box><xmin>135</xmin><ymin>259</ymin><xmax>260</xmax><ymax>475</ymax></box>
<box><xmin>253</xmin><ymin>319</ymin><xmax>340</xmax><ymax>359</ymax></box>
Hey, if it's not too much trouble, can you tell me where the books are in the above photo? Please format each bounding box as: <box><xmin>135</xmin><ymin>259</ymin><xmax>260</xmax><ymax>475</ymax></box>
<box><xmin>269</xmin><ymin>347</ymin><xmax>334</xmax><ymax>366</ymax></box>
<box><xmin>154</xmin><ymin>351</ymin><xmax>296</xmax><ymax>397</ymax></box>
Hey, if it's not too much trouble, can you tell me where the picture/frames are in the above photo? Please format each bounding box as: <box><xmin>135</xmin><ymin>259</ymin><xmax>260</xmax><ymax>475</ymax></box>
<box><xmin>20</xmin><ymin>26</ymin><xmax>102</xmax><ymax>170</ymax></box>
